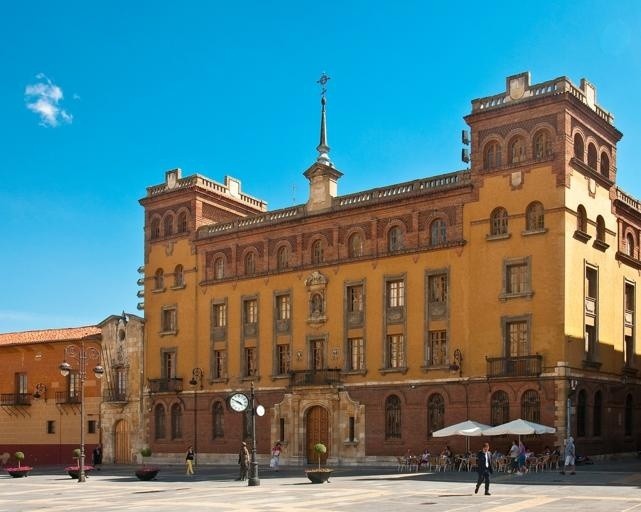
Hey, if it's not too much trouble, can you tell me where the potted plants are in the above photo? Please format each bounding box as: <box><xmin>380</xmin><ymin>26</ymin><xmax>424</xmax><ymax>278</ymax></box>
<box><xmin>64</xmin><ymin>449</ymin><xmax>94</xmax><ymax>478</ymax></box>
<box><xmin>5</xmin><ymin>451</ymin><xmax>32</xmax><ymax>479</ymax></box>
<box><xmin>133</xmin><ymin>447</ymin><xmax>159</xmax><ymax>480</ymax></box>
<box><xmin>303</xmin><ymin>443</ymin><xmax>334</xmax><ymax>484</ymax></box>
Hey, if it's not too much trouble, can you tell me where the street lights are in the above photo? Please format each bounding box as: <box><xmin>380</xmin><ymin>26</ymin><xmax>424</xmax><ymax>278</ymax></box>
<box><xmin>59</xmin><ymin>334</ymin><xmax>103</xmax><ymax>483</ymax></box>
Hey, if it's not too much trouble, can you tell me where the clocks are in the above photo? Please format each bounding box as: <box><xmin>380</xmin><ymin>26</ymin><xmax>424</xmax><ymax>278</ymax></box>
<box><xmin>227</xmin><ymin>380</ymin><xmax>259</xmax><ymax>486</ymax></box>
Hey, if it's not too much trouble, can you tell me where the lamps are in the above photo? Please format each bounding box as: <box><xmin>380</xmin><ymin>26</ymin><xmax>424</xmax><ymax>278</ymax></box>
<box><xmin>449</xmin><ymin>348</ymin><xmax>464</xmax><ymax>378</ymax></box>
<box><xmin>32</xmin><ymin>383</ymin><xmax>47</xmax><ymax>403</ymax></box>
<box><xmin>188</xmin><ymin>366</ymin><xmax>205</xmax><ymax>391</ymax></box>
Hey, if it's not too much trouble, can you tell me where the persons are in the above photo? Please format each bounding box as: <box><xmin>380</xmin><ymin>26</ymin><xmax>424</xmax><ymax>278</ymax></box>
<box><xmin>237</xmin><ymin>441</ymin><xmax>250</xmax><ymax>481</ymax></box>
<box><xmin>405</xmin><ymin>446</ymin><xmax>452</xmax><ymax>470</ymax></box>
<box><xmin>476</xmin><ymin>443</ymin><xmax>494</xmax><ymax>495</ymax></box>
<box><xmin>506</xmin><ymin>440</ymin><xmax>534</xmax><ymax>475</ymax></box>
<box><xmin>184</xmin><ymin>446</ymin><xmax>195</xmax><ymax>474</ymax></box>
<box><xmin>545</xmin><ymin>438</ymin><xmax>576</xmax><ymax>474</ymax></box>
<box><xmin>92</xmin><ymin>445</ymin><xmax>103</xmax><ymax>471</ymax></box>
<box><xmin>271</xmin><ymin>443</ymin><xmax>282</xmax><ymax>472</ymax></box>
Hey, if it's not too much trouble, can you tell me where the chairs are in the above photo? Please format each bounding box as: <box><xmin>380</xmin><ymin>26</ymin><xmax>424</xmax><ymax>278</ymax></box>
<box><xmin>492</xmin><ymin>454</ymin><xmax>559</xmax><ymax>476</ymax></box>
<box><xmin>396</xmin><ymin>453</ymin><xmax>478</xmax><ymax>473</ymax></box>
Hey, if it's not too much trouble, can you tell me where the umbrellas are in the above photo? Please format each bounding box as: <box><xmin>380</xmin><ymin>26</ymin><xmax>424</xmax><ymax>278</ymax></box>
<box><xmin>433</xmin><ymin>419</ymin><xmax>495</xmax><ymax>452</ymax></box>
<box><xmin>483</xmin><ymin>418</ymin><xmax>557</xmax><ymax>448</ymax></box>
